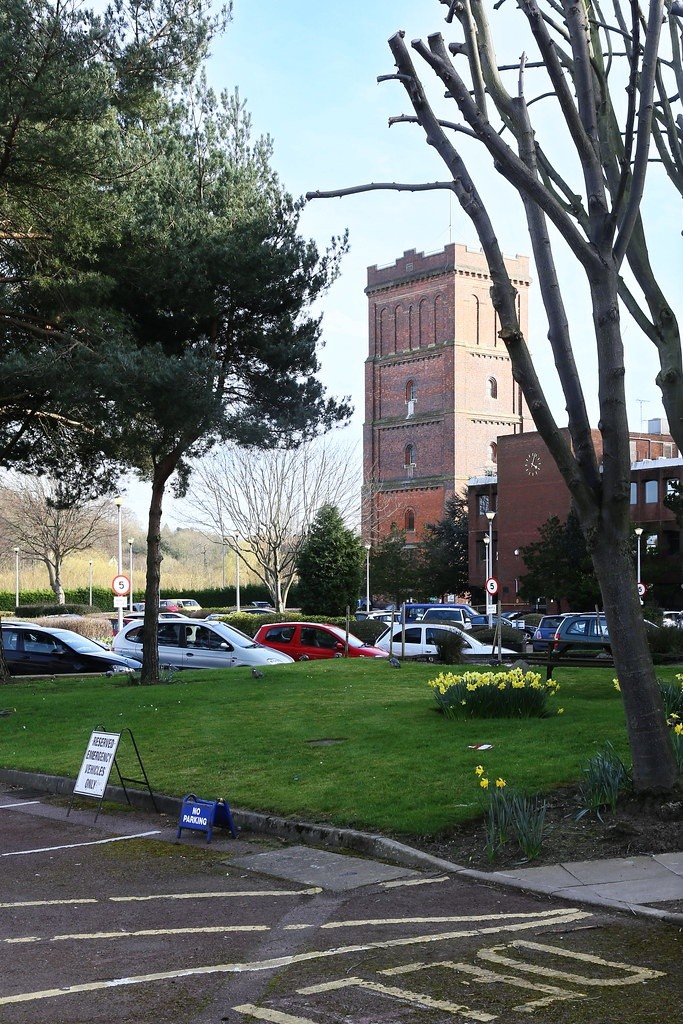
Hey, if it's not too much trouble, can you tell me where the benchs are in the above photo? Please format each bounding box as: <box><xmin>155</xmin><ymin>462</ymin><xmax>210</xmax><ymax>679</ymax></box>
<box><xmin>523</xmin><ymin>639</ymin><xmax>616</xmax><ymax>682</ymax></box>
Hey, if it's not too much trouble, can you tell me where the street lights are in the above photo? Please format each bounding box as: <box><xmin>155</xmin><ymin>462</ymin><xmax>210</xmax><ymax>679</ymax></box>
<box><xmin>485</xmin><ymin>511</ymin><xmax>497</xmax><ymax>630</ymax></box>
<box><xmin>113</xmin><ymin>494</ymin><xmax>127</xmax><ymax>633</ymax></box>
<box><xmin>365</xmin><ymin>542</ymin><xmax>371</xmax><ymax>613</ymax></box>
<box><xmin>88</xmin><ymin>558</ymin><xmax>93</xmax><ymax>606</ymax></box>
<box><xmin>14</xmin><ymin>547</ymin><xmax>20</xmax><ymax>607</ymax></box>
<box><xmin>233</xmin><ymin>528</ymin><xmax>242</xmax><ymax>612</ymax></box>
<box><xmin>483</xmin><ymin>533</ymin><xmax>491</xmax><ymax>616</ymax></box>
<box><xmin>127</xmin><ymin>536</ymin><xmax>134</xmax><ymax>612</ymax></box>
<box><xmin>634</xmin><ymin>527</ymin><xmax>644</xmax><ymax>584</ymax></box>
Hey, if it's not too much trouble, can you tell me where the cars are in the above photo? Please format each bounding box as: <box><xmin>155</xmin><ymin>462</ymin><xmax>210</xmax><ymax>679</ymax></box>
<box><xmin>561</xmin><ymin>613</ymin><xmax>609</xmax><ymax>635</ymax></box>
<box><xmin>1</xmin><ymin>627</ymin><xmax>143</xmax><ymax>675</ymax></box>
<box><xmin>496</xmin><ymin>611</ymin><xmax>536</xmax><ymax>621</ymax></box>
<box><xmin>250</xmin><ymin>601</ymin><xmax>273</xmax><ymax>608</ymax></box>
<box><xmin>415</xmin><ymin>608</ymin><xmax>473</xmax><ymax>631</ymax></box>
<box><xmin>662</xmin><ymin>611</ymin><xmax>683</xmax><ymax>629</ymax></box>
<box><xmin>365</xmin><ymin>612</ymin><xmax>423</xmax><ymax>627</ymax></box>
<box><xmin>355</xmin><ymin>610</ymin><xmax>399</xmax><ymax>621</ymax></box>
<box><xmin>469</xmin><ymin>614</ymin><xmax>538</xmax><ymax>645</ymax></box>
<box><xmin>0</xmin><ymin>622</ymin><xmax>41</xmax><ymax>647</ymax></box>
<box><xmin>230</xmin><ymin>608</ymin><xmax>277</xmax><ymax>615</ymax></box>
<box><xmin>44</xmin><ymin>598</ymin><xmax>203</xmax><ymax>636</ymax></box>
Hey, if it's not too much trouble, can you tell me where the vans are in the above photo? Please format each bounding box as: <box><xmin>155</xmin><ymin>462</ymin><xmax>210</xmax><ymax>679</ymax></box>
<box><xmin>399</xmin><ymin>604</ymin><xmax>487</xmax><ymax>627</ymax></box>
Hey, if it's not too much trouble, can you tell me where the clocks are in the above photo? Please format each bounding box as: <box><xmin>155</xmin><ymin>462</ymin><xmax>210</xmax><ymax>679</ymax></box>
<box><xmin>524</xmin><ymin>453</ymin><xmax>541</xmax><ymax>476</ymax></box>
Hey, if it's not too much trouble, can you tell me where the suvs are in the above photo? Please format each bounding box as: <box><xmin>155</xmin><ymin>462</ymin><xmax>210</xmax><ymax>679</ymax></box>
<box><xmin>533</xmin><ymin>615</ymin><xmax>566</xmax><ymax>651</ymax></box>
<box><xmin>374</xmin><ymin>624</ymin><xmax>518</xmax><ymax>658</ymax></box>
<box><xmin>253</xmin><ymin>621</ymin><xmax>390</xmax><ymax>662</ymax></box>
<box><xmin>112</xmin><ymin>619</ymin><xmax>296</xmax><ymax>672</ymax></box>
<box><xmin>551</xmin><ymin>612</ymin><xmax>661</xmax><ymax>659</ymax></box>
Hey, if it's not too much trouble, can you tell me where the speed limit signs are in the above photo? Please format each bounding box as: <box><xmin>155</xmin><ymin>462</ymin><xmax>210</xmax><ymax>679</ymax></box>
<box><xmin>637</xmin><ymin>583</ymin><xmax>646</xmax><ymax>595</ymax></box>
<box><xmin>485</xmin><ymin>578</ymin><xmax>500</xmax><ymax>596</ymax></box>
<box><xmin>111</xmin><ymin>575</ymin><xmax>130</xmax><ymax>595</ymax></box>
